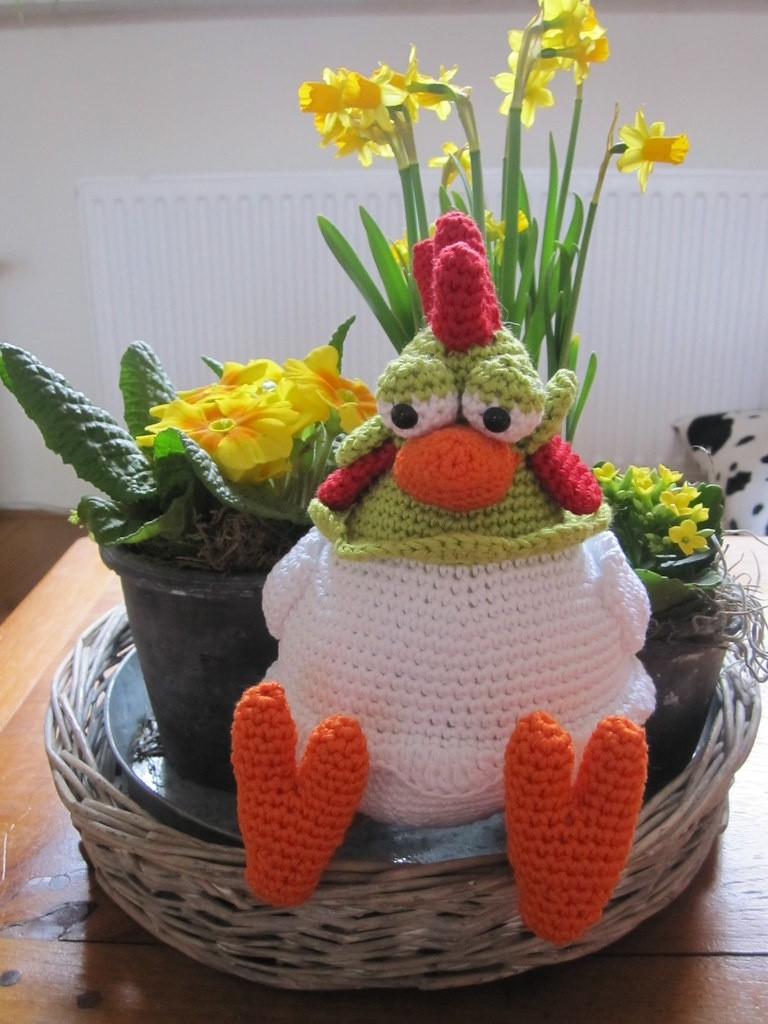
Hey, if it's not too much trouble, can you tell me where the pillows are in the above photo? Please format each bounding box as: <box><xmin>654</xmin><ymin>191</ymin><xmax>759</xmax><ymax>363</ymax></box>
<box><xmin>672</xmin><ymin>407</ymin><xmax>768</xmax><ymax>535</ymax></box>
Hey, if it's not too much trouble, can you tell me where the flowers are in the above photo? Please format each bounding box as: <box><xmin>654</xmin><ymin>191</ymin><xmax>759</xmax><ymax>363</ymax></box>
<box><xmin>0</xmin><ymin>305</ymin><xmax>382</xmax><ymax>573</ymax></box>
<box><xmin>592</xmin><ymin>458</ymin><xmax>725</xmax><ymax>614</ymax></box>
<box><xmin>298</xmin><ymin>0</ymin><xmax>691</xmax><ymax>455</ymax></box>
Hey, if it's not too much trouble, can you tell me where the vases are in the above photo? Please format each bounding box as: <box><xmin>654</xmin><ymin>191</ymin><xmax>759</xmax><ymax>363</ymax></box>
<box><xmin>99</xmin><ymin>545</ymin><xmax>292</xmax><ymax>794</ymax></box>
<box><xmin>630</xmin><ymin>638</ymin><xmax>726</xmax><ymax>793</ymax></box>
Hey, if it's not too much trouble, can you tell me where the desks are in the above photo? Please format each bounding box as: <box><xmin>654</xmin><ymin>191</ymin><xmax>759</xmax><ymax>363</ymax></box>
<box><xmin>0</xmin><ymin>536</ymin><xmax>768</xmax><ymax>1024</ymax></box>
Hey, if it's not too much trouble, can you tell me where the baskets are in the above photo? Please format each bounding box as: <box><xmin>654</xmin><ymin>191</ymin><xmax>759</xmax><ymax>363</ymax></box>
<box><xmin>44</xmin><ymin>601</ymin><xmax>762</xmax><ymax>992</ymax></box>
